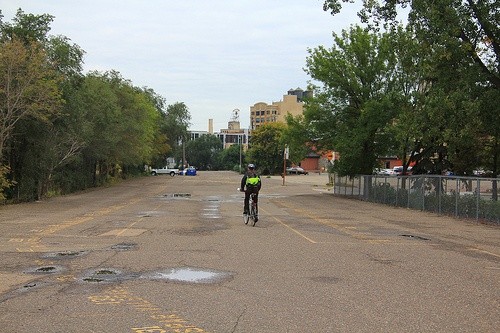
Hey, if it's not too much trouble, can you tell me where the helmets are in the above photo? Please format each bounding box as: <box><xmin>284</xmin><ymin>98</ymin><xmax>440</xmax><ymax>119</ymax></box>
<box><xmin>248</xmin><ymin>164</ymin><xmax>255</xmax><ymax>168</ymax></box>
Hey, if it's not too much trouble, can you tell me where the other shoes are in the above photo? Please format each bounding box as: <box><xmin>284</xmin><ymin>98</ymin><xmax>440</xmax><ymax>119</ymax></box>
<box><xmin>243</xmin><ymin>212</ymin><xmax>247</xmax><ymax>215</ymax></box>
<box><xmin>255</xmin><ymin>216</ymin><xmax>258</xmax><ymax>223</ymax></box>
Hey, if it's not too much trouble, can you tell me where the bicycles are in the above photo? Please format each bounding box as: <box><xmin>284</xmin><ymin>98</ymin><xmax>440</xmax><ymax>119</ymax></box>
<box><xmin>237</xmin><ymin>188</ymin><xmax>258</xmax><ymax>228</ymax></box>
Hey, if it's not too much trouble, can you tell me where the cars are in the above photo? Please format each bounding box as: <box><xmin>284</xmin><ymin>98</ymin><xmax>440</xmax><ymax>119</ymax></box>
<box><xmin>286</xmin><ymin>168</ymin><xmax>308</xmax><ymax>175</ymax></box>
<box><xmin>394</xmin><ymin>166</ymin><xmax>493</xmax><ymax>178</ymax></box>
<box><xmin>379</xmin><ymin>169</ymin><xmax>395</xmax><ymax>177</ymax></box>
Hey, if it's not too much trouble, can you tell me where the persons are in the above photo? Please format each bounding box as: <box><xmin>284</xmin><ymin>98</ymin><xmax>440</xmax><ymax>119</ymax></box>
<box><xmin>239</xmin><ymin>164</ymin><xmax>262</xmax><ymax>223</ymax></box>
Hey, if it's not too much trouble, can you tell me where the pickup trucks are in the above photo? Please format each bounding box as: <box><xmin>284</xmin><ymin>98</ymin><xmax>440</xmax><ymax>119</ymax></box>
<box><xmin>150</xmin><ymin>166</ymin><xmax>179</xmax><ymax>177</ymax></box>
